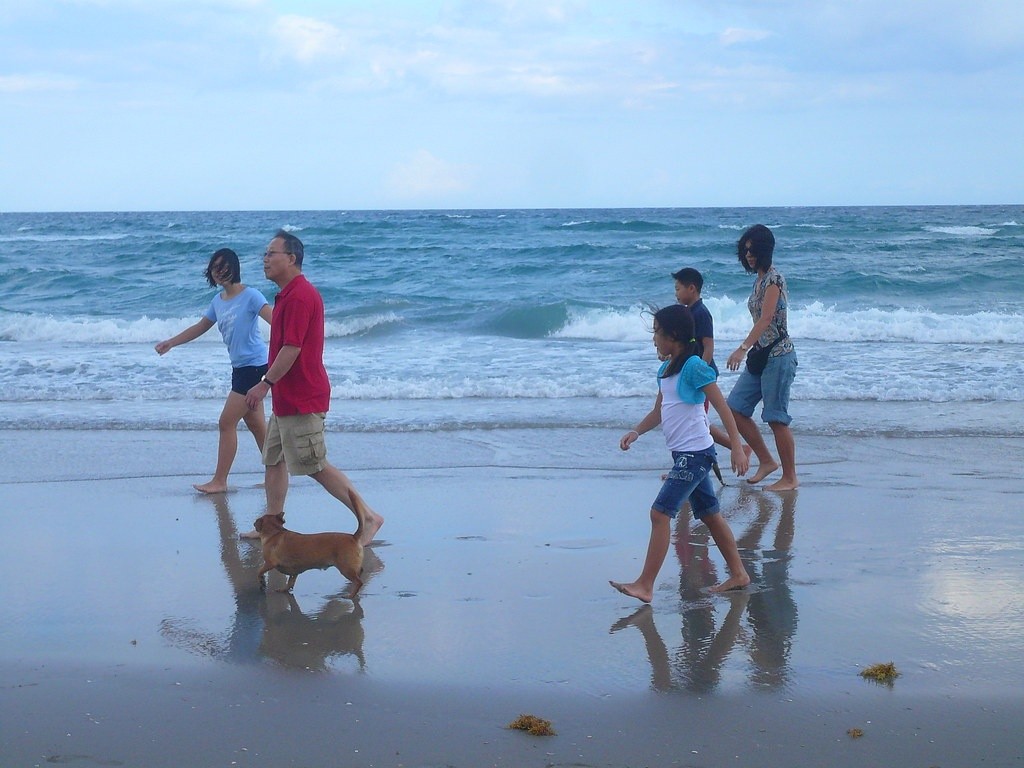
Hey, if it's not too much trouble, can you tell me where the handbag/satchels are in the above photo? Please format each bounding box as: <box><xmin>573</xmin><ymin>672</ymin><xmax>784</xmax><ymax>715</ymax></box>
<box><xmin>745</xmin><ymin>331</ymin><xmax>788</xmax><ymax>376</ymax></box>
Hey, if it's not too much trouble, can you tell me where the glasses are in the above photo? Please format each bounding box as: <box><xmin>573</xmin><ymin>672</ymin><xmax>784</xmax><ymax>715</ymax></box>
<box><xmin>264</xmin><ymin>250</ymin><xmax>292</xmax><ymax>257</ymax></box>
<box><xmin>742</xmin><ymin>245</ymin><xmax>754</xmax><ymax>257</ymax></box>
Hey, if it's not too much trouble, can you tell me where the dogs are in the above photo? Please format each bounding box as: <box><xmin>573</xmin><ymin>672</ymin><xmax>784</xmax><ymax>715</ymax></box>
<box><xmin>254</xmin><ymin>487</ymin><xmax>366</xmax><ymax>599</ymax></box>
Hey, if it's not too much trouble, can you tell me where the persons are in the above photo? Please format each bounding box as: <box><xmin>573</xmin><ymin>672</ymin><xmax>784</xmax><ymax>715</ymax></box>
<box><xmin>662</xmin><ymin>267</ymin><xmax>753</xmax><ymax>478</ymax></box>
<box><xmin>239</xmin><ymin>229</ymin><xmax>384</xmax><ymax>551</ymax></box>
<box><xmin>608</xmin><ymin>479</ymin><xmax>797</xmax><ymax>697</ymax></box>
<box><xmin>153</xmin><ymin>248</ymin><xmax>273</xmax><ymax>492</ymax></box>
<box><xmin>724</xmin><ymin>224</ymin><xmax>801</xmax><ymax>490</ymax></box>
<box><xmin>606</xmin><ymin>304</ymin><xmax>754</xmax><ymax>602</ymax></box>
<box><xmin>164</xmin><ymin>491</ymin><xmax>270</xmax><ymax>663</ymax></box>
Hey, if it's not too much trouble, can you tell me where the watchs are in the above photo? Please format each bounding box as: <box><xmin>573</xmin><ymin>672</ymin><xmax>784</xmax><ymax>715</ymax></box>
<box><xmin>261</xmin><ymin>375</ymin><xmax>274</xmax><ymax>387</ymax></box>
<box><xmin>741</xmin><ymin>344</ymin><xmax>748</xmax><ymax>351</ymax></box>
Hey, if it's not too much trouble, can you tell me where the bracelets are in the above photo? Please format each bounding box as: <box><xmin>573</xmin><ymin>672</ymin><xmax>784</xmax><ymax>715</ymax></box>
<box><xmin>630</xmin><ymin>431</ymin><xmax>640</xmax><ymax>436</ymax></box>
